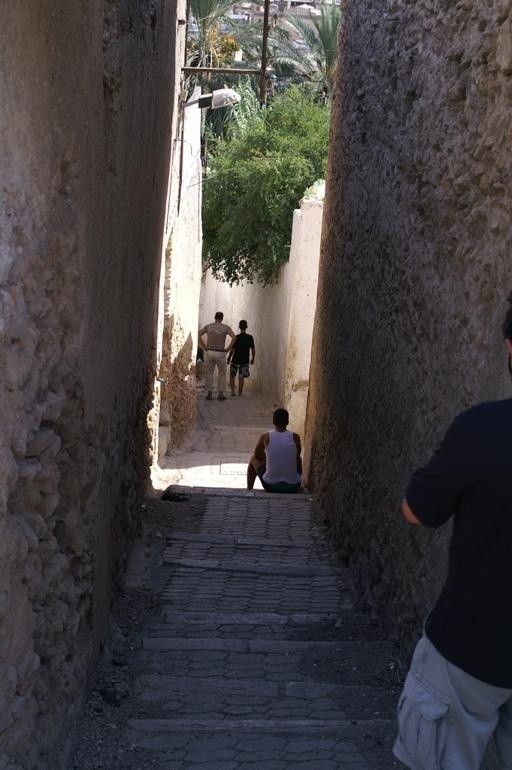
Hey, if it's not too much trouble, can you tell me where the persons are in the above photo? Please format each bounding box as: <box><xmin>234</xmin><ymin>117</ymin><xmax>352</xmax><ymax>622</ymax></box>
<box><xmin>227</xmin><ymin>320</ymin><xmax>255</xmax><ymax>398</ymax></box>
<box><xmin>198</xmin><ymin>312</ymin><xmax>236</xmax><ymax>401</ymax></box>
<box><xmin>389</xmin><ymin>307</ymin><xmax>512</xmax><ymax>770</ymax></box>
<box><xmin>245</xmin><ymin>408</ymin><xmax>303</xmax><ymax>494</ymax></box>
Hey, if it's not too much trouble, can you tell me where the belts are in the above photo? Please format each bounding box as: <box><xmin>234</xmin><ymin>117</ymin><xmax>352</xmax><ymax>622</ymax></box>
<box><xmin>207</xmin><ymin>348</ymin><xmax>225</xmax><ymax>352</ymax></box>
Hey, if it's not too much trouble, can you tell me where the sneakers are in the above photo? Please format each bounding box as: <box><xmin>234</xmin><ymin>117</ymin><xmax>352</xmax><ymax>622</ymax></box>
<box><xmin>232</xmin><ymin>394</ymin><xmax>236</xmax><ymax>396</ymax></box>
<box><xmin>205</xmin><ymin>395</ymin><xmax>212</xmax><ymax>400</ymax></box>
<box><xmin>218</xmin><ymin>397</ymin><xmax>226</xmax><ymax>401</ymax></box>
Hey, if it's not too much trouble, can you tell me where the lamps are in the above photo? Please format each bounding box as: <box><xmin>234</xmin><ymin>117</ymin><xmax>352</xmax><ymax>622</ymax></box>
<box><xmin>185</xmin><ymin>87</ymin><xmax>243</xmax><ymax>110</ymax></box>
